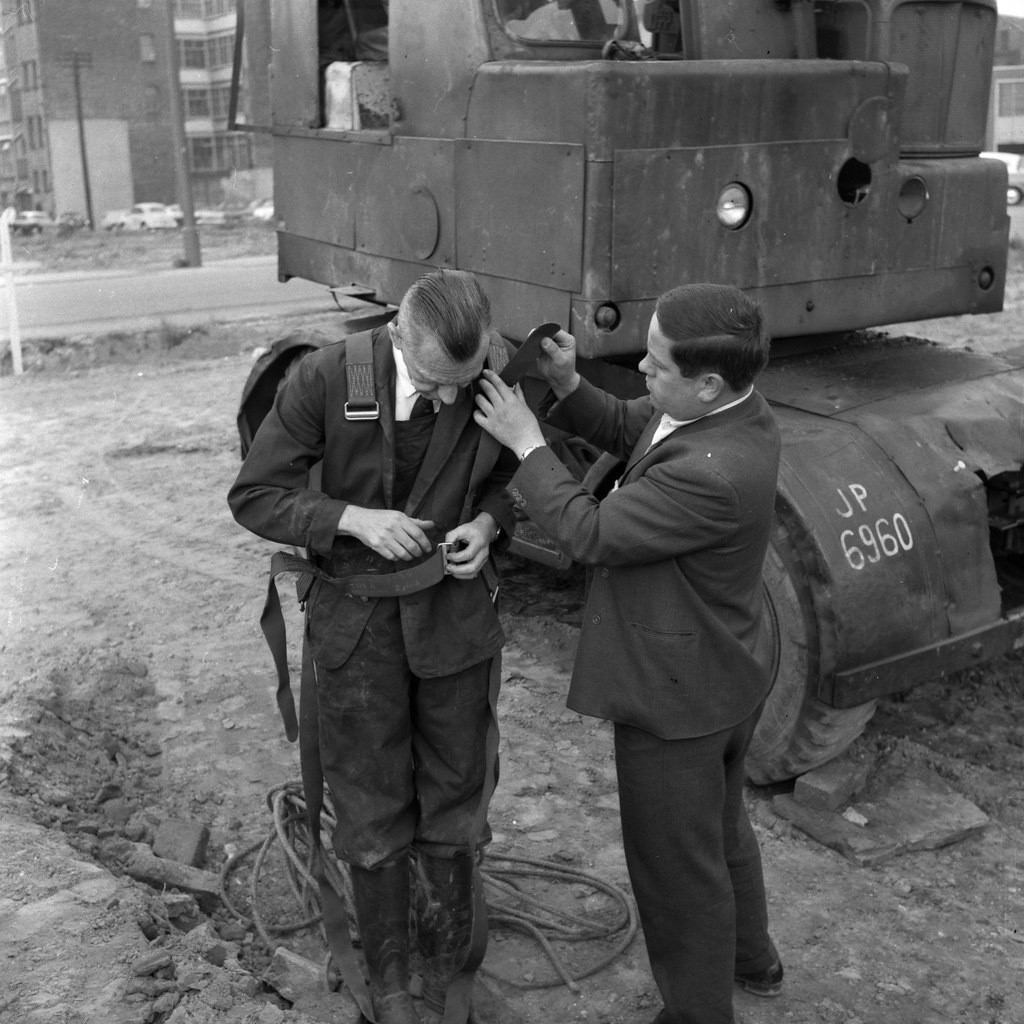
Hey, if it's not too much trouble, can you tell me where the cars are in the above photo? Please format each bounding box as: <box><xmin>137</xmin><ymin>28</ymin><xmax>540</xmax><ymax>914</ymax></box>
<box><xmin>57</xmin><ymin>210</ymin><xmax>92</xmax><ymax>239</ymax></box>
<box><xmin>10</xmin><ymin>210</ymin><xmax>54</xmax><ymax>235</ymax></box>
<box><xmin>101</xmin><ymin>195</ymin><xmax>274</xmax><ymax>230</ymax></box>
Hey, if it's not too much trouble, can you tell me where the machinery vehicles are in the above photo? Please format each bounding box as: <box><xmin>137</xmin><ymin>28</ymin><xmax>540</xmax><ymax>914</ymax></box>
<box><xmin>223</xmin><ymin>1</ymin><xmax>1024</xmax><ymax>789</ymax></box>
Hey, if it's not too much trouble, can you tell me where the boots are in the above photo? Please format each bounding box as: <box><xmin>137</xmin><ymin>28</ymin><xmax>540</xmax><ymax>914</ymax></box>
<box><xmin>413</xmin><ymin>853</ymin><xmax>483</xmax><ymax>1024</ymax></box>
<box><xmin>350</xmin><ymin>857</ymin><xmax>423</xmax><ymax>1024</ymax></box>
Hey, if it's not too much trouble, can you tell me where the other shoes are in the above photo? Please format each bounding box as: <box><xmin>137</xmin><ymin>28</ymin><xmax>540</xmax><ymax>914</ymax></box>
<box><xmin>733</xmin><ymin>956</ymin><xmax>784</xmax><ymax>997</ymax></box>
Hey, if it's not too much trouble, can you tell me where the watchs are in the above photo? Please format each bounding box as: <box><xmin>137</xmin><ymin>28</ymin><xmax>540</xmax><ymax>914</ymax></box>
<box><xmin>519</xmin><ymin>446</ymin><xmax>538</xmax><ymax>463</ymax></box>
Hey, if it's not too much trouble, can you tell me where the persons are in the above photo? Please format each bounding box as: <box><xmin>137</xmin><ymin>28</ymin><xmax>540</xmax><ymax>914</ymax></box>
<box><xmin>227</xmin><ymin>272</ymin><xmax>602</xmax><ymax>1022</ymax></box>
<box><xmin>473</xmin><ymin>284</ymin><xmax>784</xmax><ymax>1023</ymax></box>
